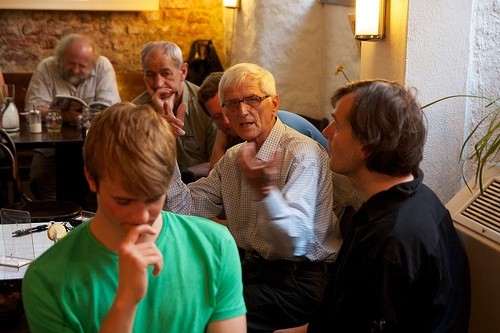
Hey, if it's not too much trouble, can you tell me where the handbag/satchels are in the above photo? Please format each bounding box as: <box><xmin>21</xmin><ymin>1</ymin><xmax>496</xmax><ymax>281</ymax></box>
<box><xmin>186</xmin><ymin>39</ymin><xmax>224</xmax><ymax>87</ymax></box>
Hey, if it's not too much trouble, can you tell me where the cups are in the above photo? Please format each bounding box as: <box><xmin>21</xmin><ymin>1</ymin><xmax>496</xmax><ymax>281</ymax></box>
<box><xmin>82</xmin><ymin>108</ymin><xmax>101</xmax><ymax>138</ymax></box>
<box><xmin>29</xmin><ymin>110</ymin><xmax>42</xmax><ymax>133</ymax></box>
<box><xmin>2</xmin><ymin>84</ymin><xmax>15</xmax><ymax>104</ymax></box>
<box><xmin>46</xmin><ymin>111</ymin><xmax>63</xmax><ymax>133</ymax></box>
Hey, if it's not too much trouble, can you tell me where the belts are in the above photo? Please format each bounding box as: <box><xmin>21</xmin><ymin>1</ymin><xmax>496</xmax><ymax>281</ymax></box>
<box><xmin>259</xmin><ymin>260</ymin><xmax>324</xmax><ymax>271</ymax></box>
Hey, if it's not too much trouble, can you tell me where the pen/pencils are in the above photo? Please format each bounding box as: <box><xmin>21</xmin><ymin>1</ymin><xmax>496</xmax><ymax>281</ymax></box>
<box><xmin>12</xmin><ymin>224</ymin><xmax>48</xmax><ymax>235</ymax></box>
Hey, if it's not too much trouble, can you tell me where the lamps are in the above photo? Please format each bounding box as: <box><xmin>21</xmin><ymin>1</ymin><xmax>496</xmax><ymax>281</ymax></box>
<box><xmin>355</xmin><ymin>0</ymin><xmax>385</xmax><ymax>39</ymax></box>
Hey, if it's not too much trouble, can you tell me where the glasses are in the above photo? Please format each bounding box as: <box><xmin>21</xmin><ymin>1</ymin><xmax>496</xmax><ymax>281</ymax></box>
<box><xmin>221</xmin><ymin>95</ymin><xmax>269</xmax><ymax>111</ymax></box>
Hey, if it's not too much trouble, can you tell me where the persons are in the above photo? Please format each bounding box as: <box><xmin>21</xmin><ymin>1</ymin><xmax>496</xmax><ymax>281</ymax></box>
<box><xmin>22</xmin><ymin>34</ymin><xmax>331</xmax><ymax>228</ymax></box>
<box><xmin>163</xmin><ymin>64</ymin><xmax>343</xmax><ymax>333</ymax></box>
<box><xmin>22</xmin><ymin>102</ymin><xmax>248</xmax><ymax>333</ymax></box>
<box><xmin>274</xmin><ymin>79</ymin><xmax>472</xmax><ymax>333</ymax></box>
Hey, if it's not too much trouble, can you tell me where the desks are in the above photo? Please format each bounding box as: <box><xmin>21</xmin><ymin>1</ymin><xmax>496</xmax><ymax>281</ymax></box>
<box><xmin>0</xmin><ymin>121</ymin><xmax>98</xmax><ymax>333</ymax></box>
